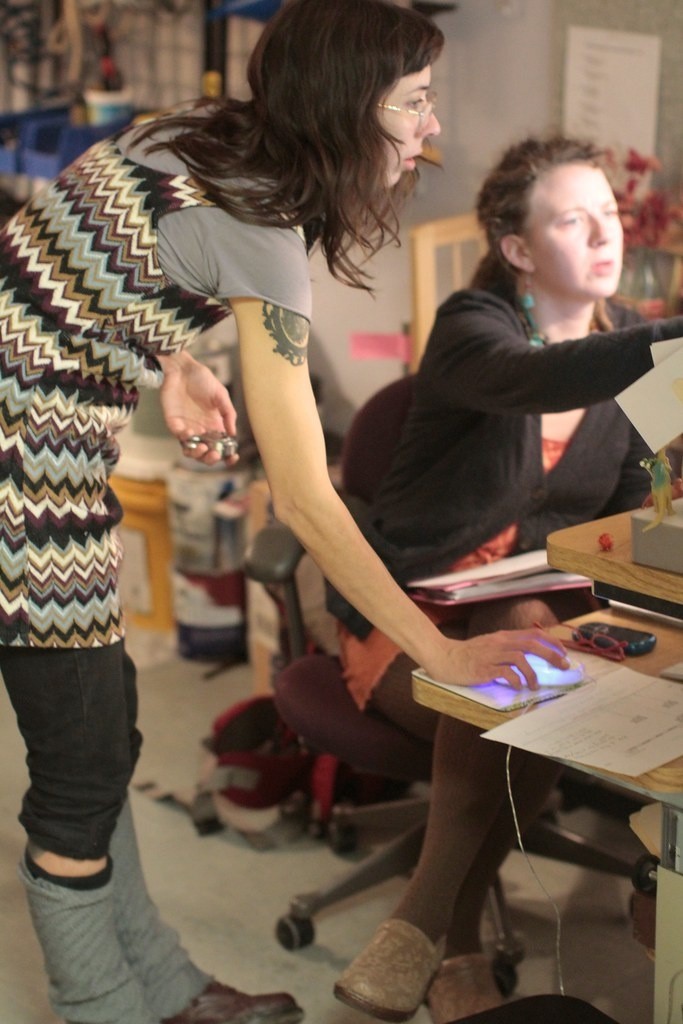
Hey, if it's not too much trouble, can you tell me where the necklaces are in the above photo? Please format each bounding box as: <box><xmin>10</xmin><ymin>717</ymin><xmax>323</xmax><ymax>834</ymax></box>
<box><xmin>512</xmin><ymin>295</ymin><xmax>546</xmax><ymax>348</ymax></box>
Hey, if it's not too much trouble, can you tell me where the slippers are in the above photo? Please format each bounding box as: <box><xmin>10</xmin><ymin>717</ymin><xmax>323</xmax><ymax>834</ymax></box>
<box><xmin>333</xmin><ymin>917</ymin><xmax>507</xmax><ymax>1024</ymax></box>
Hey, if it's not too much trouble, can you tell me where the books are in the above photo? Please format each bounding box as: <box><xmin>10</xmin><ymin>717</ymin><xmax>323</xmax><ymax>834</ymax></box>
<box><xmin>405</xmin><ymin>549</ymin><xmax>593</xmax><ymax>605</ymax></box>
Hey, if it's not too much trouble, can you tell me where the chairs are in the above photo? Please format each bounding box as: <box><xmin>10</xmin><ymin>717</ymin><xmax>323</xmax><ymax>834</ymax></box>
<box><xmin>270</xmin><ymin>373</ymin><xmax>663</xmax><ymax>1001</ymax></box>
<box><xmin>408</xmin><ymin>209</ymin><xmax>488</xmax><ymax>375</ymax></box>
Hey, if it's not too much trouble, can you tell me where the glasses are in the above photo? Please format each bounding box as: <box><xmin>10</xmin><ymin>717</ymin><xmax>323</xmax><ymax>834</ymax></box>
<box><xmin>377</xmin><ymin>90</ymin><xmax>440</xmax><ymax>129</ymax></box>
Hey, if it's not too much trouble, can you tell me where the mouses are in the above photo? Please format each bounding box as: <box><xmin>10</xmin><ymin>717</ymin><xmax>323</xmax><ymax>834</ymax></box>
<box><xmin>494</xmin><ymin>643</ymin><xmax>585</xmax><ymax>688</ymax></box>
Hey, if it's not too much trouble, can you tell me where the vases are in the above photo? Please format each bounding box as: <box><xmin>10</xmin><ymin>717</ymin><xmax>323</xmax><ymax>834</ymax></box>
<box><xmin>627</xmin><ymin>247</ymin><xmax>669</xmax><ymax>323</ymax></box>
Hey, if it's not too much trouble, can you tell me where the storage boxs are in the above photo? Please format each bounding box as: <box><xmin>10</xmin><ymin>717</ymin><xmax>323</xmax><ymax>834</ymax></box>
<box><xmin>104</xmin><ymin>444</ymin><xmax>181</xmax><ymax>636</ymax></box>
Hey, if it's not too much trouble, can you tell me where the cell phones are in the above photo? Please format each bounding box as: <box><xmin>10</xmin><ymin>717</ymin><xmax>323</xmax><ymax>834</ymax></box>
<box><xmin>573</xmin><ymin>622</ymin><xmax>658</xmax><ymax>656</ymax></box>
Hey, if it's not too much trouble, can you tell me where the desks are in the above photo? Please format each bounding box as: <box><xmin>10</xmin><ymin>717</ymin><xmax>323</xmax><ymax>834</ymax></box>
<box><xmin>412</xmin><ymin>493</ymin><xmax>683</xmax><ymax>1024</ymax></box>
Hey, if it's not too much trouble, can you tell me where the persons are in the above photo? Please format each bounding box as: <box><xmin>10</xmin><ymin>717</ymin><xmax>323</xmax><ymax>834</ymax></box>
<box><xmin>321</xmin><ymin>136</ymin><xmax>682</xmax><ymax>1024</ymax></box>
<box><xmin>2</xmin><ymin>0</ymin><xmax>572</xmax><ymax>1023</ymax></box>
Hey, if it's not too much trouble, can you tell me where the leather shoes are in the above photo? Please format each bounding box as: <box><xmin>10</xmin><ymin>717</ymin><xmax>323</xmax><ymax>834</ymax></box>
<box><xmin>159</xmin><ymin>980</ymin><xmax>303</xmax><ymax>1024</ymax></box>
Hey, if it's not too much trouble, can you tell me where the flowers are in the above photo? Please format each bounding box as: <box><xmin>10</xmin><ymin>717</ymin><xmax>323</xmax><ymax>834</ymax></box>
<box><xmin>609</xmin><ymin>145</ymin><xmax>680</xmax><ymax>262</ymax></box>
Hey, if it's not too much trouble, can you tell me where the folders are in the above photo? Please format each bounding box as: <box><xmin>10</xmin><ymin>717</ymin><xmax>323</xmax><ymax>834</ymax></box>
<box><xmin>408</xmin><ymin>549</ymin><xmax>591</xmax><ymax>606</ymax></box>
